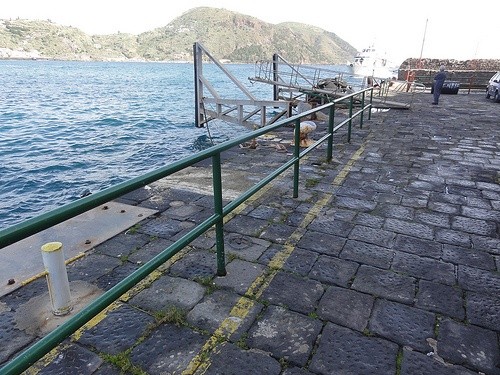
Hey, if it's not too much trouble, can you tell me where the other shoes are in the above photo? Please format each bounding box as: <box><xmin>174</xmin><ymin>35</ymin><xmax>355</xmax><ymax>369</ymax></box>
<box><xmin>432</xmin><ymin>102</ymin><xmax>438</xmax><ymax>105</ymax></box>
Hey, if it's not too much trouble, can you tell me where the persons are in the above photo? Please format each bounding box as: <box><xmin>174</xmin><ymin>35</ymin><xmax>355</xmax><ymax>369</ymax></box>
<box><xmin>419</xmin><ymin>60</ymin><xmax>425</xmax><ymax>68</ymax></box>
<box><xmin>449</xmin><ymin>71</ymin><xmax>457</xmax><ymax>81</ymax></box>
<box><xmin>408</xmin><ymin>70</ymin><xmax>415</xmax><ymax>82</ymax></box>
<box><xmin>432</xmin><ymin>65</ymin><xmax>447</xmax><ymax>105</ymax></box>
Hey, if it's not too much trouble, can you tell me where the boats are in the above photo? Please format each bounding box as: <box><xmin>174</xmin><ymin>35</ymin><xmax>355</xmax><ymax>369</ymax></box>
<box><xmin>349</xmin><ymin>38</ymin><xmax>393</xmax><ymax>80</ymax></box>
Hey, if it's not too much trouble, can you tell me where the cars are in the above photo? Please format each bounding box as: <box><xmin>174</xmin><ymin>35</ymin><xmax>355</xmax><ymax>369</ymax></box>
<box><xmin>430</xmin><ymin>70</ymin><xmax>461</xmax><ymax>95</ymax></box>
<box><xmin>485</xmin><ymin>70</ymin><xmax>500</xmax><ymax>103</ymax></box>
